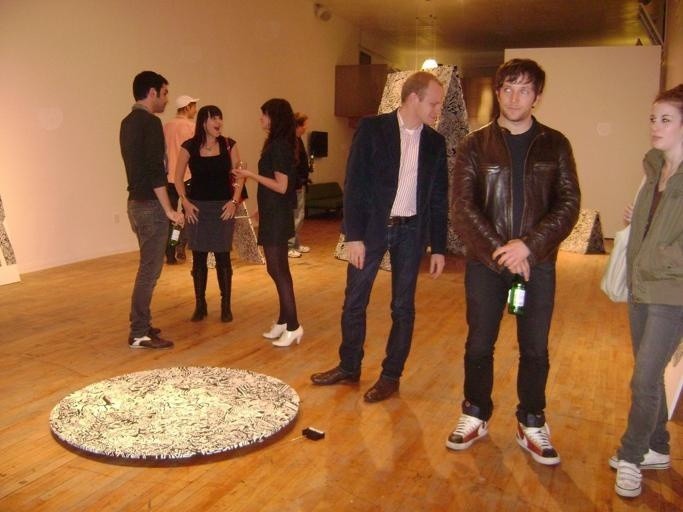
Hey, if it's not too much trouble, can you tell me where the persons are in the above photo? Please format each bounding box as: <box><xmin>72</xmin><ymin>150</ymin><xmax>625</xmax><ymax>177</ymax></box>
<box><xmin>446</xmin><ymin>59</ymin><xmax>581</xmax><ymax>464</ymax></box>
<box><xmin>610</xmin><ymin>84</ymin><xmax>683</xmax><ymax>500</ymax></box>
<box><xmin>310</xmin><ymin>73</ymin><xmax>450</xmax><ymax>403</ymax></box>
<box><xmin>232</xmin><ymin>99</ymin><xmax>303</xmax><ymax>348</ymax></box>
<box><xmin>286</xmin><ymin>113</ymin><xmax>314</xmax><ymax>258</ymax></box>
<box><xmin>174</xmin><ymin>106</ymin><xmax>244</xmax><ymax>323</ymax></box>
<box><xmin>166</xmin><ymin>96</ymin><xmax>200</xmax><ymax>270</ymax></box>
<box><xmin>121</xmin><ymin>71</ymin><xmax>182</xmax><ymax>351</ymax></box>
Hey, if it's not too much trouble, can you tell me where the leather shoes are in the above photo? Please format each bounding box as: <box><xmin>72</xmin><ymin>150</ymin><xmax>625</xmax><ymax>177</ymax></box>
<box><xmin>311</xmin><ymin>367</ymin><xmax>362</xmax><ymax>386</ymax></box>
<box><xmin>363</xmin><ymin>379</ymin><xmax>400</xmax><ymax>403</ymax></box>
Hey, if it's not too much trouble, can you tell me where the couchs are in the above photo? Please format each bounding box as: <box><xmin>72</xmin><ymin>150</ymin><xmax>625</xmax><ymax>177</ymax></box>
<box><xmin>306</xmin><ymin>182</ymin><xmax>344</xmax><ymax>218</ymax></box>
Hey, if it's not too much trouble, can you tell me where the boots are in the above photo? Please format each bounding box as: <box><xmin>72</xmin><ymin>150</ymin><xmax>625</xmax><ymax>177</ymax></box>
<box><xmin>216</xmin><ymin>266</ymin><xmax>235</xmax><ymax>323</ymax></box>
<box><xmin>190</xmin><ymin>267</ymin><xmax>208</xmax><ymax>322</ymax></box>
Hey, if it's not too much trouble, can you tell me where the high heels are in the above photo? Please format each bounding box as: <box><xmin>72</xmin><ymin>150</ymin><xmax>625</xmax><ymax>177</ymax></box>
<box><xmin>263</xmin><ymin>323</ymin><xmax>287</xmax><ymax>339</ymax></box>
<box><xmin>271</xmin><ymin>324</ymin><xmax>304</xmax><ymax>348</ymax></box>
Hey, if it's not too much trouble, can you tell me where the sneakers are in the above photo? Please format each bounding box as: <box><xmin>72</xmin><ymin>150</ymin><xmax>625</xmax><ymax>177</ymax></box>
<box><xmin>127</xmin><ymin>323</ymin><xmax>162</xmax><ymax>335</ymax></box>
<box><xmin>608</xmin><ymin>447</ymin><xmax>671</xmax><ymax>472</ymax></box>
<box><xmin>614</xmin><ymin>462</ymin><xmax>644</xmax><ymax>499</ymax></box>
<box><xmin>128</xmin><ymin>330</ymin><xmax>174</xmax><ymax>351</ymax></box>
<box><xmin>288</xmin><ymin>248</ymin><xmax>301</xmax><ymax>257</ymax></box>
<box><xmin>514</xmin><ymin>420</ymin><xmax>561</xmax><ymax>467</ymax></box>
<box><xmin>297</xmin><ymin>245</ymin><xmax>310</xmax><ymax>253</ymax></box>
<box><xmin>445</xmin><ymin>413</ymin><xmax>489</xmax><ymax>452</ymax></box>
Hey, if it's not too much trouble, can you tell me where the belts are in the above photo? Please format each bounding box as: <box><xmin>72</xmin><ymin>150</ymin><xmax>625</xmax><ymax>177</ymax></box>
<box><xmin>387</xmin><ymin>213</ymin><xmax>417</xmax><ymax>225</ymax></box>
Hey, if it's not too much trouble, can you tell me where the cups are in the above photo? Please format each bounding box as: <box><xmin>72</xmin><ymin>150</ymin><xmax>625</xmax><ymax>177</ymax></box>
<box><xmin>235</xmin><ymin>160</ymin><xmax>247</xmax><ymax>184</ymax></box>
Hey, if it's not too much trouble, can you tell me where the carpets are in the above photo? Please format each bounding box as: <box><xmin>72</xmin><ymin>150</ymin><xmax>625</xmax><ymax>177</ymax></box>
<box><xmin>51</xmin><ymin>367</ymin><xmax>301</xmax><ymax>461</ymax></box>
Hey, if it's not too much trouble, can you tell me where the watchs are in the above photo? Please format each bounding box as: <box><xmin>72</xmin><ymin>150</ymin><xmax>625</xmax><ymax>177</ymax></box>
<box><xmin>231</xmin><ymin>198</ymin><xmax>240</xmax><ymax>210</ymax></box>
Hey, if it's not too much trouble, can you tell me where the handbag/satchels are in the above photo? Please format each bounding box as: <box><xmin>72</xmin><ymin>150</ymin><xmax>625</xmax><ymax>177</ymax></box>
<box><xmin>225</xmin><ymin>137</ymin><xmax>249</xmax><ymax>204</ymax></box>
<box><xmin>601</xmin><ymin>175</ymin><xmax>648</xmax><ymax>303</ymax></box>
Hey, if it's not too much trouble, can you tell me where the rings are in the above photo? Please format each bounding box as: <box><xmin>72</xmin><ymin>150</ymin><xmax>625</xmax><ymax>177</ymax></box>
<box><xmin>190</xmin><ymin>216</ymin><xmax>192</xmax><ymax>218</ymax></box>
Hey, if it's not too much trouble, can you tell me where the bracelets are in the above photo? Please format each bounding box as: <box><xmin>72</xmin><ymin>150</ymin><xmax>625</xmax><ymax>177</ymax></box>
<box><xmin>179</xmin><ymin>195</ymin><xmax>186</xmax><ymax>202</ymax></box>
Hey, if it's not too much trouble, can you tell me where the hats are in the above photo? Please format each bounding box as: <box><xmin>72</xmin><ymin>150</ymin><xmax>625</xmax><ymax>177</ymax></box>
<box><xmin>176</xmin><ymin>95</ymin><xmax>201</xmax><ymax>110</ymax></box>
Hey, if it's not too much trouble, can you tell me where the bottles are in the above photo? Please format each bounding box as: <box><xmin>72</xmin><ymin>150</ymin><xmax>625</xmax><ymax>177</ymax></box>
<box><xmin>169</xmin><ymin>223</ymin><xmax>182</xmax><ymax>247</ymax></box>
<box><xmin>507</xmin><ymin>274</ymin><xmax>526</xmax><ymax>317</ymax></box>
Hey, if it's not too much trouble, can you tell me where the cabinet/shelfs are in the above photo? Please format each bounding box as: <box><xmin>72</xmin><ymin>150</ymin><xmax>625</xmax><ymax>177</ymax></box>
<box><xmin>335</xmin><ymin>65</ymin><xmax>394</xmax><ymax>117</ymax></box>
